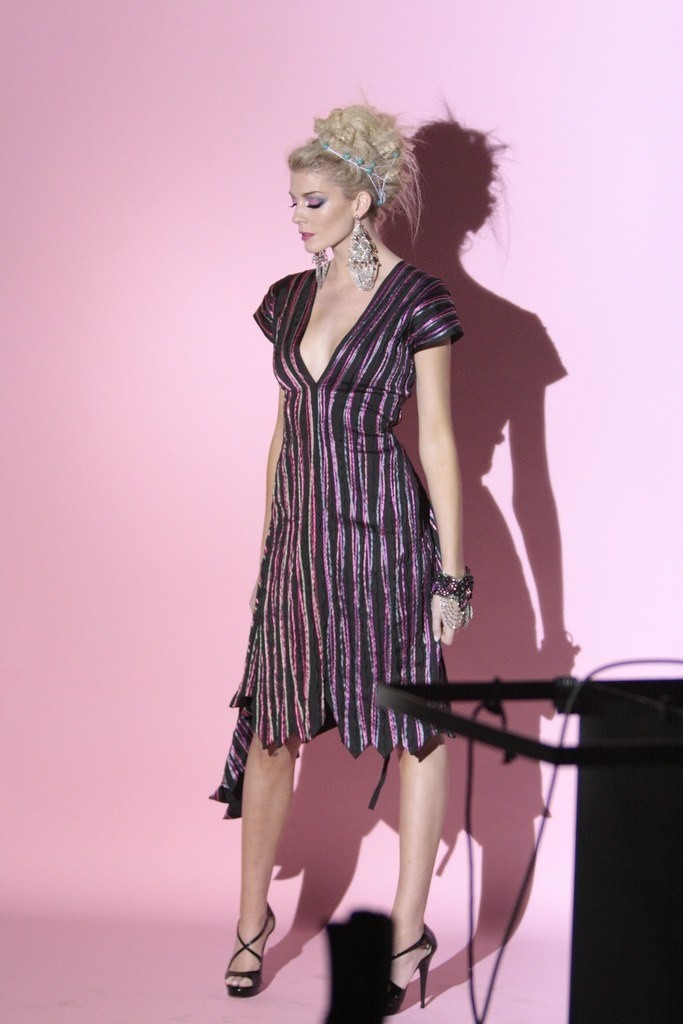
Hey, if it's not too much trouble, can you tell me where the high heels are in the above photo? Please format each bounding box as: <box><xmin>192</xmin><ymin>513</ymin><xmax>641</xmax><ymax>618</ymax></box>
<box><xmin>225</xmin><ymin>902</ymin><xmax>276</xmax><ymax>997</ymax></box>
<box><xmin>385</xmin><ymin>923</ymin><xmax>437</xmax><ymax>1016</ymax></box>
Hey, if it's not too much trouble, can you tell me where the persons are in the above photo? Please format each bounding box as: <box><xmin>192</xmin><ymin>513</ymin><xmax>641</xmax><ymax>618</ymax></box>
<box><xmin>209</xmin><ymin>105</ymin><xmax>475</xmax><ymax>1012</ymax></box>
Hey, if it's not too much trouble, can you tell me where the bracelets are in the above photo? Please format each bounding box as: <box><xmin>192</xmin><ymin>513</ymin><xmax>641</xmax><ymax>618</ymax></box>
<box><xmin>432</xmin><ymin>570</ymin><xmax>473</xmax><ymax>629</ymax></box>
